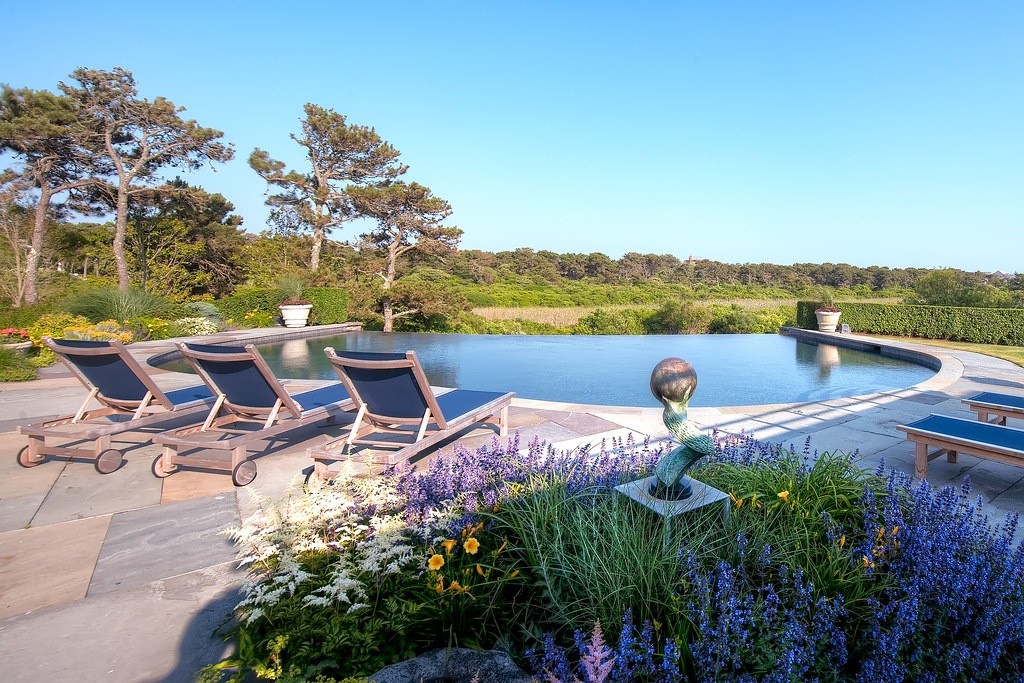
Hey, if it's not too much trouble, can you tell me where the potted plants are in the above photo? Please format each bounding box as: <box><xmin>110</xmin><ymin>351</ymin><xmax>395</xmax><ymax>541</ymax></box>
<box><xmin>278</xmin><ymin>277</ymin><xmax>313</xmax><ymax>328</ymax></box>
<box><xmin>815</xmin><ymin>289</ymin><xmax>842</xmax><ymax>333</ymax></box>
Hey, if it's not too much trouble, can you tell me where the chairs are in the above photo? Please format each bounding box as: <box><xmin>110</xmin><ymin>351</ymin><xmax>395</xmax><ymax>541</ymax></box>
<box><xmin>961</xmin><ymin>391</ymin><xmax>1024</xmax><ymax>426</ymax></box>
<box><xmin>151</xmin><ymin>340</ymin><xmax>359</xmax><ymax>486</ymax></box>
<box><xmin>16</xmin><ymin>335</ymin><xmax>223</xmax><ymax>474</ymax></box>
<box><xmin>896</xmin><ymin>413</ymin><xmax>1024</xmax><ymax>481</ymax></box>
<box><xmin>303</xmin><ymin>346</ymin><xmax>517</xmax><ymax>497</ymax></box>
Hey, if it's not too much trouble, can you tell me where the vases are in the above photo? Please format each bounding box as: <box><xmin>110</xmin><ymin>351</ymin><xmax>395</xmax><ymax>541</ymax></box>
<box><xmin>0</xmin><ymin>341</ymin><xmax>32</xmax><ymax>354</ymax></box>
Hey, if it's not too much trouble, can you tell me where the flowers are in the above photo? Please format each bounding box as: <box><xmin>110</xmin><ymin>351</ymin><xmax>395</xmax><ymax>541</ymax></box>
<box><xmin>0</xmin><ymin>327</ymin><xmax>30</xmax><ymax>343</ymax></box>
